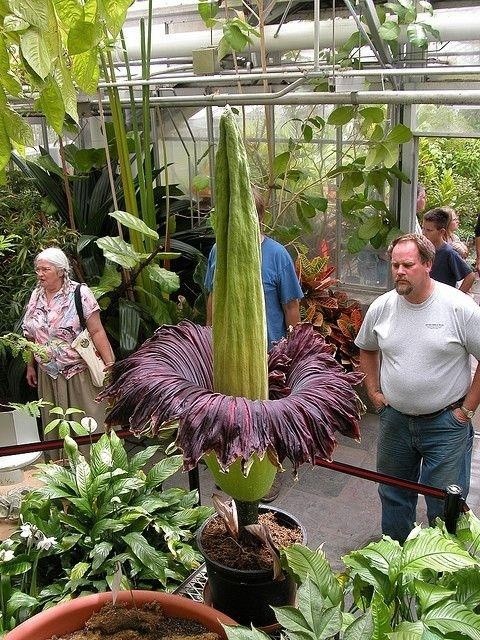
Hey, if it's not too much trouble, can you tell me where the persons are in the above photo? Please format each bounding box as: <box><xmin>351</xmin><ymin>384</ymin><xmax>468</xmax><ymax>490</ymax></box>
<box><xmin>203</xmin><ymin>188</ymin><xmax>303</xmax><ymax>504</ymax></box>
<box><xmin>315</xmin><ymin>182</ymin><xmax>480</xmax><ymax>293</ymax></box>
<box><xmin>21</xmin><ymin>245</ymin><xmax>118</xmax><ymax>465</ymax></box>
<box><xmin>353</xmin><ymin>232</ymin><xmax>480</xmax><ymax>547</ymax></box>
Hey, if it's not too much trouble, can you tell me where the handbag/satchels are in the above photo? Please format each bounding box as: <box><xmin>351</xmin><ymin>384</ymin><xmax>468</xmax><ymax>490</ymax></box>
<box><xmin>69</xmin><ymin>284</ymin><xmax>116</xmax><ymax>388</ymax></box>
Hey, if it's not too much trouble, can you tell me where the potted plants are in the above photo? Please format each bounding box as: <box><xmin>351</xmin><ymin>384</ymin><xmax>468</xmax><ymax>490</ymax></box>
<box><xmin>93</xmin><ymin>109</ymin><xmax>364</xmax><ymax>640</ymax></box>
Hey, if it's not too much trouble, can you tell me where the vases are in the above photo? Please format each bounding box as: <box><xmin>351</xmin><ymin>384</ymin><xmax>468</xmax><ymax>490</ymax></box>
<box><xmin>2</xmin><ymin>589</ymin><xmax>240</xmax><ymax>640</ymax></box>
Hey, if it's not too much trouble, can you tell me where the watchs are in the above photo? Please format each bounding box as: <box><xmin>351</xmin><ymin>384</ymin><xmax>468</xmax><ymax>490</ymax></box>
<box><xmin>459</xmin><ymin>406</ymin><xmax>475</xmax><ymax>419</ymax></box>
